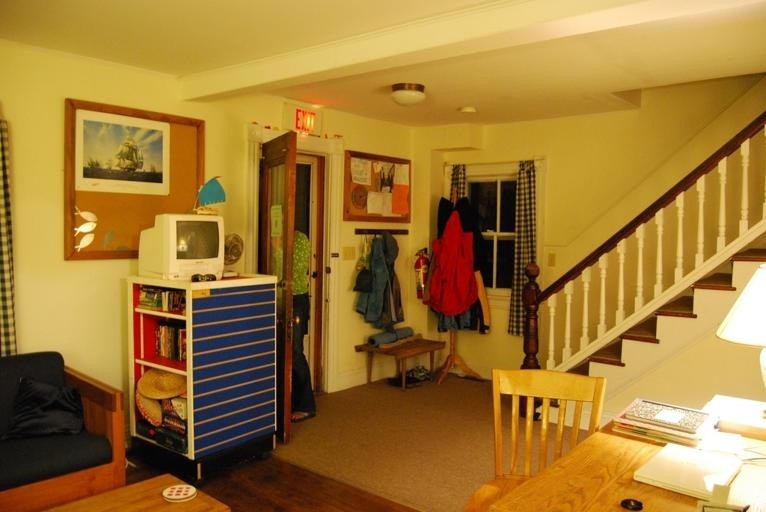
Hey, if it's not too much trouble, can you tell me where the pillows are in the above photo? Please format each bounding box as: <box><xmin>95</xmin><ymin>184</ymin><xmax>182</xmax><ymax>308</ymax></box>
<box><xmin>0</xmin><ymin>374</ymin><xmax>85</xmax><ymax>443</ymax></box>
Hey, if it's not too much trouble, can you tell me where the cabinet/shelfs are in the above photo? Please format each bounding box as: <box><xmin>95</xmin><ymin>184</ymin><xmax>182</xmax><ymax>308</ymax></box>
<box><xmin>123</xmin><ymin>272</ymin><xmax>280</xmax><ymax>483</ymax></box>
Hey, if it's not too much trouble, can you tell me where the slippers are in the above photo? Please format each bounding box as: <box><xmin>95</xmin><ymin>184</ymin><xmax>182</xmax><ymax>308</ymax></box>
<box><xmin>291</xmin><ymin>412</ymin><xmax>315</xmax><ymax>423</ymax></box>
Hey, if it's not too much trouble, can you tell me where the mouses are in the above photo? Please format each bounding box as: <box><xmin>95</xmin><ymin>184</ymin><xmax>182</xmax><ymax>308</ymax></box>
<box><xmin>621</xmin><ymin>499</ymin><xmax>643</xmax><ymax>511</ymax></box>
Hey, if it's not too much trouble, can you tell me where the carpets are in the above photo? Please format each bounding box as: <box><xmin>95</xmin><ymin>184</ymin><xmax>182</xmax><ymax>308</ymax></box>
<box><xmin>267</xmin><ymin>370</ymin><xmax>593</xmax><ymax>510</ymax></box>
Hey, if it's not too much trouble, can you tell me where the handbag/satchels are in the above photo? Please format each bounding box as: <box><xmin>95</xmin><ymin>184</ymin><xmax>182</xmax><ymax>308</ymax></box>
<box><xmin>353</xmin><ymin>269</ymin><xmax>373</xmax><ymax>292</ymax></box>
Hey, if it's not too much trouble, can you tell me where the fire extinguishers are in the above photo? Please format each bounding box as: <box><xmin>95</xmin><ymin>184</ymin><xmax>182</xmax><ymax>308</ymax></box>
<box><xmin>414</xmin><ymin>247</ymin><xmax>430</xmax><ymax>299</ymax></box>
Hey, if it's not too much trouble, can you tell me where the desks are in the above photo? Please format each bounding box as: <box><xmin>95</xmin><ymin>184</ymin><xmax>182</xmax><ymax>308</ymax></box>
<box><xmin>480</xmin><ymin>412</ymin><xmax>766</xmax><ymax>512</ymax></box>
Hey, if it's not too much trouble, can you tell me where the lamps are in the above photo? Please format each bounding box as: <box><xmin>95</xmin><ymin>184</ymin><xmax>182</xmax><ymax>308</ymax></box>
<box><xmin>714</xmin><ymin>261</ymin><xmax>766</xmax><ymax>400</ymax></box>
<box><xmin>390</xmin><ymin>81</ymin><xmax>428</xmax><ymax>106</ymax></box>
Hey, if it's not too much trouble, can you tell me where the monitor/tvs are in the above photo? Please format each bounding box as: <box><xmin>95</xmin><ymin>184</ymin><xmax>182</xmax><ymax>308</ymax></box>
<box><xmin>139</xmin><ymin>214</ymin><xmax>224</xmax><ymax>280</ymax></box>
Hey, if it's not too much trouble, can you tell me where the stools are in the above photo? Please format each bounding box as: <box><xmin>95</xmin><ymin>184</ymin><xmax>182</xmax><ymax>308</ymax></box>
<box><xmin>354</xmin><ymin>338</ymin><xmax>447</xmax><ymax>393</ymax></box>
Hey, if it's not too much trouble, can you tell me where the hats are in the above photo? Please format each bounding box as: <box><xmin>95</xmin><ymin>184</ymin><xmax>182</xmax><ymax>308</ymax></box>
<box><xmin>138</xmin><ymin>367</ymin><xmax>187</xmax><ymax>400</ymax></box>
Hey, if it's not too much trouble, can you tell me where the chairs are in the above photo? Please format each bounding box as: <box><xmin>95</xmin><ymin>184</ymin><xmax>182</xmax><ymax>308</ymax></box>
<box><xmin>460</xmin><ymin>365</ymin><xmax>609</xmax><ymax>511</ymax></box>
<box><xmin>0</xmin><ymin>350</ymin><xmax>129</xmax><ymax>511</ymax></box>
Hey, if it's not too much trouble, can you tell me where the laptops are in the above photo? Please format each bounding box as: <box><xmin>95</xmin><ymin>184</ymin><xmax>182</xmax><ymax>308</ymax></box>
<box><xmin>635</xmin><ymin>442</ymin><xmax>743</xmax><ymax>501</ymax></box>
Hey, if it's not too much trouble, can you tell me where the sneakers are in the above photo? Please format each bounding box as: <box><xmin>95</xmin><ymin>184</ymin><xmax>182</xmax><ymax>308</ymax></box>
<box><xmin>389</xmin><ymin>365</ymin><xmax>431</xmax><ymax>387</ymax></box>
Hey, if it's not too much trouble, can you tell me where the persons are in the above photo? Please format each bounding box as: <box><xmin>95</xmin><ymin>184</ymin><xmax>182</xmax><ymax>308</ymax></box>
<box><xmin>272</xmin><ymin>229</ymin><xmax>315</xmax><ymax>422</ymax></box>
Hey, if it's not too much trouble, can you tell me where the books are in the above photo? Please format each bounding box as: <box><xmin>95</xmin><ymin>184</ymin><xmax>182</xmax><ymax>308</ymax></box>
<box><xmin>136</xmin><ymin>286</ymin><xmax>187</xmax><ymax>362</ymax></box>
<box><xmin>612</xmin><ymin>398</ymin><xmax>743</xmax><ymax>502</ymax></box>
